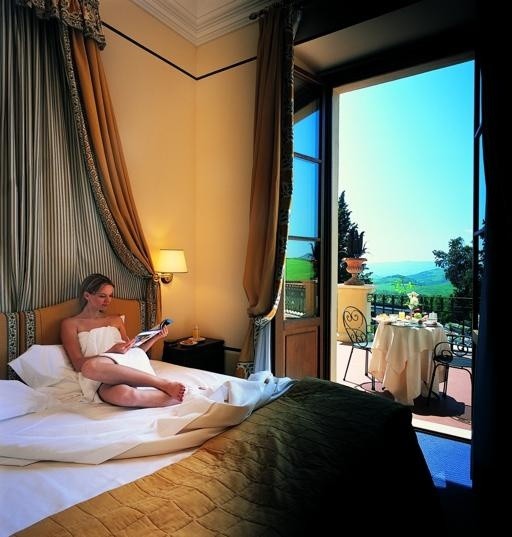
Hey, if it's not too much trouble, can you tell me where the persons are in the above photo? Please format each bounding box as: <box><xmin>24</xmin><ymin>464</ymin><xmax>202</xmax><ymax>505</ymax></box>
<box><xmin>60</xmin><ymin>272</ymin><xmax>187</xmax><ymax>409</ymax></box>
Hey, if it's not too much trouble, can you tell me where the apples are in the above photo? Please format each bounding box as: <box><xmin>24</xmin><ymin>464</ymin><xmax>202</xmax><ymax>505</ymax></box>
<box><xmin>408</xmin><ymin>307</ymin><xmax>421</xmax><ymax>319</ymax></box>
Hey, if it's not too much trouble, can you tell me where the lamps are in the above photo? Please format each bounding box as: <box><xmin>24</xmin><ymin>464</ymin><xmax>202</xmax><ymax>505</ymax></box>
<box><xmin>158</xmin><ymin>250</ymin><xmax>188</xmax><ymax>283</ymax></box>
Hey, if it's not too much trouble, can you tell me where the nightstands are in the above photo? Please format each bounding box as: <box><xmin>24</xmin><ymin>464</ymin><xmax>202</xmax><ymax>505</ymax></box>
<box><xmin>162</xmin><ymin>336</ymin><xmax>226</xmax><ymax>375</ymax></box>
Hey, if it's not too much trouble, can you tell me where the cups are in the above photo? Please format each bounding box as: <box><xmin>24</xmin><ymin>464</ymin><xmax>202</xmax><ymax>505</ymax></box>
<box><xmin>389</xmin><ymin>312</ymin><xmax>438</xmax><ymax>327</ymax></box>
<box><xmin>192</xmin><ymin>323</ymin><xmax>201</xmax><ymax>339</ymax></box>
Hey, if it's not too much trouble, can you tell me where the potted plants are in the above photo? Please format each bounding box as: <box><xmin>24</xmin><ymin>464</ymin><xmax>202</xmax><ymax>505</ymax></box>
<box><xmin>338</xmin><ymin>226</ymin><xmax>367</xmax><ymax>285</ymax></box>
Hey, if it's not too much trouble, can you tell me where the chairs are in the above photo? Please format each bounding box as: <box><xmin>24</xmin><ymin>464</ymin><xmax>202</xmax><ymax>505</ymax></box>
<box><xmin>343</xmin><ymin>306</ymin><xmax>375</xmax><ymax>391</ymax></box>
<box><xmin>427</xmin><ymin>335</ymin><xmax>474</xmax><ymax>411</ymax></box>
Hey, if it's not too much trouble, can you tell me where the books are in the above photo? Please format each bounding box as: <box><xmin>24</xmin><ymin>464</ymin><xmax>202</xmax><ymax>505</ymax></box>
<box><xmin>131</xmin><ymin>318</ymin><xmax>173</xmax><ymax>348</ymax></box>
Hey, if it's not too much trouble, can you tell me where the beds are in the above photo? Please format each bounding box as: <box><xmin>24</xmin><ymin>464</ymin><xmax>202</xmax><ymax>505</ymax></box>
<box><xmin>0</xmin><ymin>297</ymin><xmax>439</xmax><ymax>537</ymax></box>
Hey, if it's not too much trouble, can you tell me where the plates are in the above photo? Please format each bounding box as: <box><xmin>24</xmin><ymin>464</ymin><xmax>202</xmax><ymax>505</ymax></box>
<box><xmin>189</xmin><ymin>337</ymin><xmax>206</xmax><ymax>341</ymax></box>
<box><xmin>372</xmin><ymin>317</ymin><xmax>398</xmax><ymax>324</ymax></box>
<box><xmin>179</xmin><ymin>341</ymin><xmax>198</xmax><ymax>346</ymax></box>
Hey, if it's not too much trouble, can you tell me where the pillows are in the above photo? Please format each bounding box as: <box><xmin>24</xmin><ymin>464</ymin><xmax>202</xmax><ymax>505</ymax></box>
<box><xmin>7</xmin><ymin>344</ymin><xmax>158</xmax><ymax>403</ymax></box>
<box><xmin>0</xmin><ymin>379</ymin><xmax>63</xmax><ymax>421</ymax></box>
